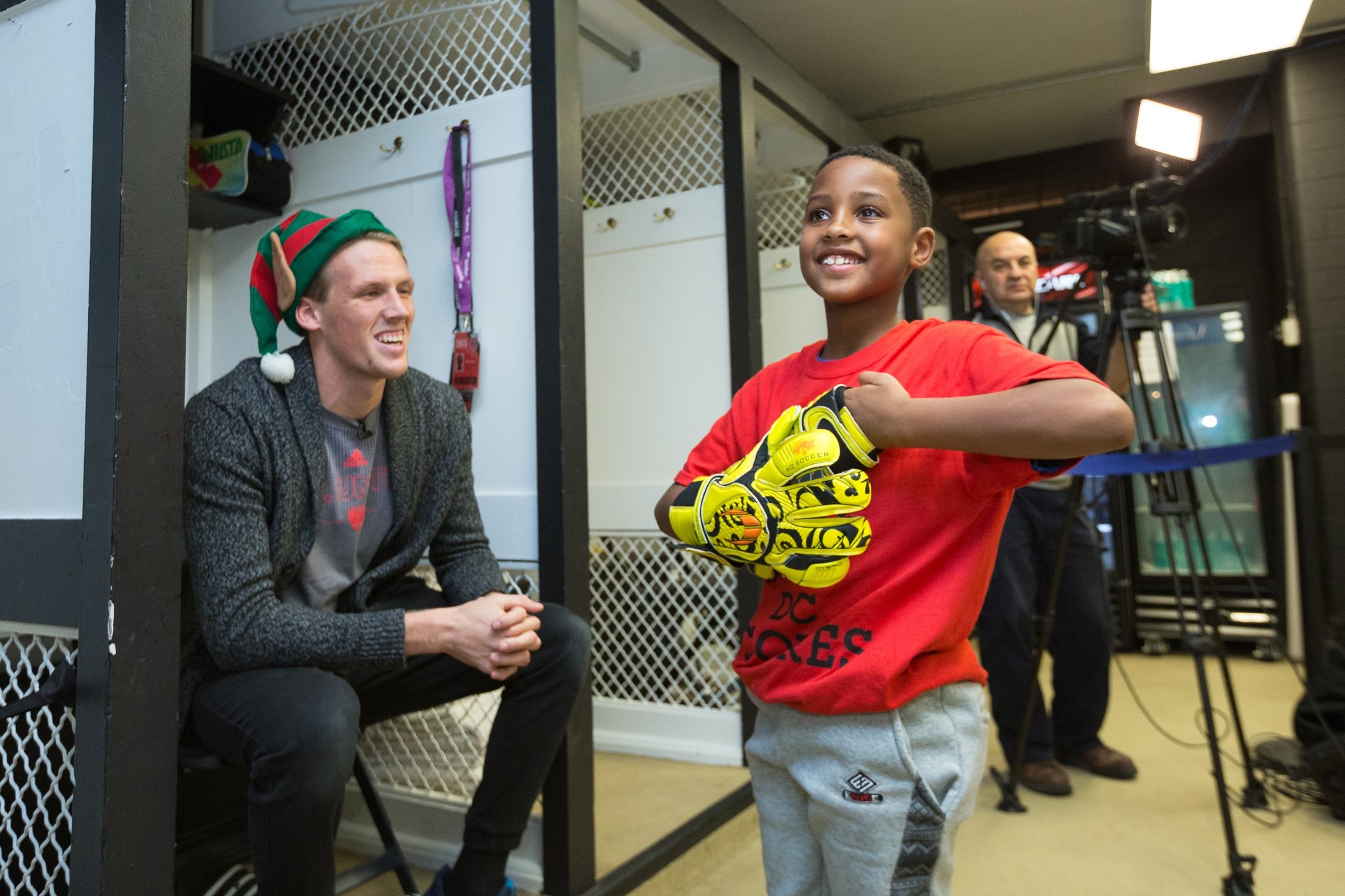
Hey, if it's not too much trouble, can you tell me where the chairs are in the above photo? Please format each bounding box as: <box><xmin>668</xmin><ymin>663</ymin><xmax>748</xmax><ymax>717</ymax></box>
<box><xmin>176</xmin><ymin>749</ymin><xmax>420</xmax><ymax>896</ymax></box>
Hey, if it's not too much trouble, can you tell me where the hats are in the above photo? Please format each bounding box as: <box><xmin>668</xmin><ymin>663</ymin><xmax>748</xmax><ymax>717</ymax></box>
<box><xmin>249</xmin><ymin>208</ymin><xmax>396</xmax><ymax>385</ymax></box>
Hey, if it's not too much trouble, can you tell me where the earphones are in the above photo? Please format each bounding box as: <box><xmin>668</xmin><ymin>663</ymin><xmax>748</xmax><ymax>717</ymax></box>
<box><xmin>980</xmin><ymin>280</ymin><xmax>985</xmax><ymax>289</ymax></box>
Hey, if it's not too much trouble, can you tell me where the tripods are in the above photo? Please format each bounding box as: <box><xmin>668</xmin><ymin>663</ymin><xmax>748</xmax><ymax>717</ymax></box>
<box><xmin>991</xmin><ymin>256</ymin><xmax>1266</xmax><ymax>896</ymax></box>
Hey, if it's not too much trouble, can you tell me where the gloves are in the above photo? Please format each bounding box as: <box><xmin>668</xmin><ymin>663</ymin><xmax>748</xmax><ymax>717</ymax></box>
<box><xmin>667</xmin><ymin>383</ymin><xmax>884</xmax><ymax>588</ymax></box>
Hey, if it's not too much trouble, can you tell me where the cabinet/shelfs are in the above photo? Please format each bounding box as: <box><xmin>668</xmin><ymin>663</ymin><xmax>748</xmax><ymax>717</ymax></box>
<box><xmin>189</xmin><ymin>54</ymin><xmax>297</xmax><ymax>229</ymax></box>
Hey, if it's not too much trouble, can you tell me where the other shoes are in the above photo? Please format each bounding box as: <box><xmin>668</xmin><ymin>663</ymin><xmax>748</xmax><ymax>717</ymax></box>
<box><xmin>1056</xmin><ymin>743</ymin><xmax>1136</xmax><ymax>779</ymax></box>
<box><xmin>1013</xmin><ymin>759</ymin><xmax>1070</xmax><ymax>793</ymax></box>
<box><xmin>421</xmin><ymin>864</ymin><xmax>518</xmax><ymax>896</ymax></box>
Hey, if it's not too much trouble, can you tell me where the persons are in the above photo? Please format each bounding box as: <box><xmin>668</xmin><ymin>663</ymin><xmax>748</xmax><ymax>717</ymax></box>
<box><xmin>654</xmin><ymin>143</ymin><xmax>1136</xmax><ymax>896</ymax></box>
<box><xmin>175</xmin><ymin>207</ymin><xmax>590</xmax><ymax>896</ymax></box>
<box><xmin>948</xmin><ymin>231</ymin><xmax>1160</xmax><ymax>797</ymax></box>
